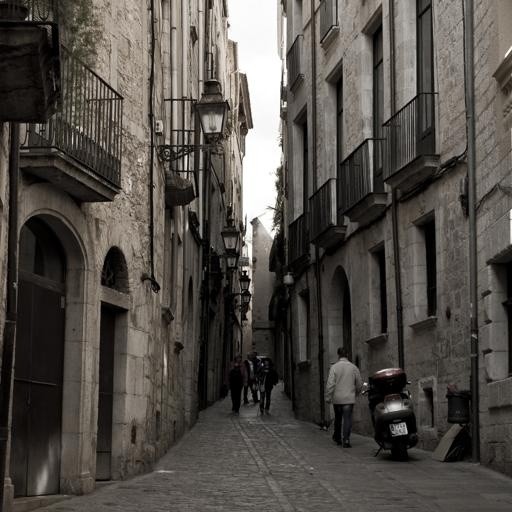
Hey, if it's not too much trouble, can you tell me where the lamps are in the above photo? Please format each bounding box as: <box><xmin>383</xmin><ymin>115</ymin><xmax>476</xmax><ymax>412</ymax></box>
<box><xmin>155</xmin><ymin>79</ymin><xmax>233</xmax><ymax>162</ymax></box>
<box><xmin>208</xmin><ymin>202</ymin><xmax>251</xmax><ymax>312</ymax></box>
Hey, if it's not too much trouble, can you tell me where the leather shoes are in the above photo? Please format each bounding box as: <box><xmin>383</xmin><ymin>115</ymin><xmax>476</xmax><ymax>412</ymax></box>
<box><xmin>332</xmin><ymin>433</ymin><xmax>350</xmax><ymax>448</ymax></box>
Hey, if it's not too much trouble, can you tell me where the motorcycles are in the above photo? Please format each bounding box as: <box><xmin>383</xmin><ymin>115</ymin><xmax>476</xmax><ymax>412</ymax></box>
<box><xmin>361</xmin><ymin>367</ymin><xmax>419</xmax><ymax>461</ymax></box>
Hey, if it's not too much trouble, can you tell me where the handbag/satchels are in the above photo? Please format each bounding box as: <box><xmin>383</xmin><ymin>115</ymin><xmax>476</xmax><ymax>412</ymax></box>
<box><xmin>257</xmin><ymin>384</ymin><xmax>265</xmax><ymax>400</ymax></box>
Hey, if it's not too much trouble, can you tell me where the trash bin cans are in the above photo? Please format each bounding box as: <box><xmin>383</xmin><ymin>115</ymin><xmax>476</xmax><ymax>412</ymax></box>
<box><xmin>446</xmin><ymin>392</ymin><xmax>468</xmax><ymax>423</ymax></box>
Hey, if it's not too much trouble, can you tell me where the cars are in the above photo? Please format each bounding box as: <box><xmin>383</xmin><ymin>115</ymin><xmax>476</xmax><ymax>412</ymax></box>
<box><xmin>255</xmin><ymin>355</ymin><xmax>279</xmax><ymax>386</ymax></box>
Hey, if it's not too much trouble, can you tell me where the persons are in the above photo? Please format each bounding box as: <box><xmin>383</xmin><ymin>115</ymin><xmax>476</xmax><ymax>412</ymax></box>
<box><xmin>325</xmin><ymin>347</ymin><xmax>363</xmax><ymax>448</ymax></box>
<box><xmin>256</xmin><ymin>358</ymin><xmax>277</xmax><ymax>417</ymax></box>
<box><xmin>241</xmin><ymin>352</ymin><xmax>260</xmax><ymax>404</ymax></box>
<box><xmin>227</xmin><ymin>363</ymin><xmax>243</xmax><ymax>413</ymax></box>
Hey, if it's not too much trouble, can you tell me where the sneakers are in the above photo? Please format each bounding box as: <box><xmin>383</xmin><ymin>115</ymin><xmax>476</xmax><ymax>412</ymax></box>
<box><xmin>260</xmin><ymin>408</ymin><xmax>271</xmax><ymax>416</ymax></box>
<box><xmin>232</xmin><ymin>410</ymin><xmax>239</xmax><ymax>416</ymax></box>
